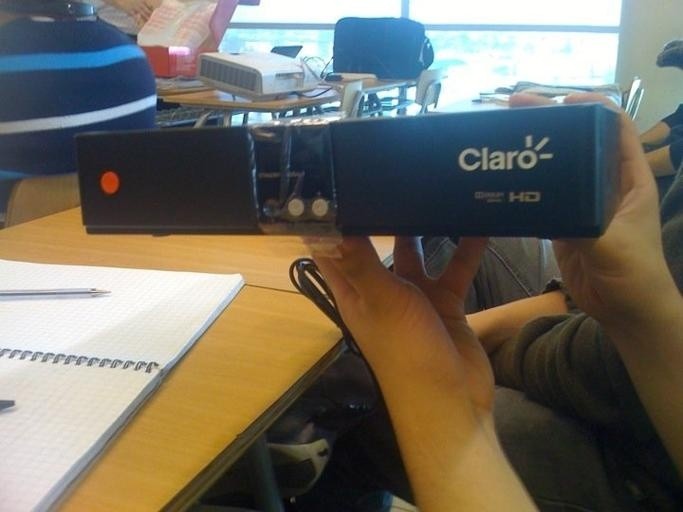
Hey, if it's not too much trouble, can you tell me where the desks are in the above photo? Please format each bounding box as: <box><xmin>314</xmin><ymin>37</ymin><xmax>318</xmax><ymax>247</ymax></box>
<box><xmin>0</xmin><ymin>183</ymin><xmax>422</xmax><ymax>511</ymax></box>
<box><xmin>152</xmin><ymin>77</ymin><xmax>221</xmax><ymax>132</ymax></box>
<box><xmin>310</xmin><ymin>70</ymin><xmax>418</xmax><ymax>117</ymax></box>
<box><xmin>162</xmin><ymin>86</ymin><xmax>339</xmax><ymax>129</ymax></box>
<box><xmin>460</xmin><ymin>81</ymin><xmax>616</xmax><ymax>108</ymax></box>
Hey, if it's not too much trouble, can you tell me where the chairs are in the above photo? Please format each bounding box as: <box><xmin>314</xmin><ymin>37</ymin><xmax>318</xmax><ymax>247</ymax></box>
<box><xmin>621</xmin><ymin>78</ymin><xmax>644</xmax><ymax>121</ymax></box>
<box><xmin>412</xmin><ymin>67</ymin><xmax>448</xmax><ymax>116</ymax></box>
<box><xmin>7</xmin><ymin>168</ymin><xmax>81</xmax><ymax>227</ymax></box>
<box><xmin>340</xmin><ymin>77</ymin><xmax>380</xmax><ymax>115</ymax></box>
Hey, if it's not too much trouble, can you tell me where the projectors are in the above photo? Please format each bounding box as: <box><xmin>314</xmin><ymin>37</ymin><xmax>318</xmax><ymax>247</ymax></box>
<box><xmin>195</xmin><ymin>51</ymin><xmax>319</xmax><ymax>97</ymax></box>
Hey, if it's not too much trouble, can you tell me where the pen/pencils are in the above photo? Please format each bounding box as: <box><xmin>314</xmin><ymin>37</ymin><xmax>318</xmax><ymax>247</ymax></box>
<box><xmin>0</xmin><ymin>287</ymin><xmax>112</xmax><ymax>300</ymax></box>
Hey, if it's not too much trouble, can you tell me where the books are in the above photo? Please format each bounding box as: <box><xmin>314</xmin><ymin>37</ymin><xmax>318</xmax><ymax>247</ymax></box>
<box><xmin>0</xmin><ymin>259</ymin><xmax>246</xmax><ymax>512</ymax></box>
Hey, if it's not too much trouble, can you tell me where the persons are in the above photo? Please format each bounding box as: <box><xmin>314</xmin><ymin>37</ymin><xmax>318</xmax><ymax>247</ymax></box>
<box><xmin>302</xmin><ymin>42</ymin><xmax>683</xmax><ymax>510</ymax></box>
